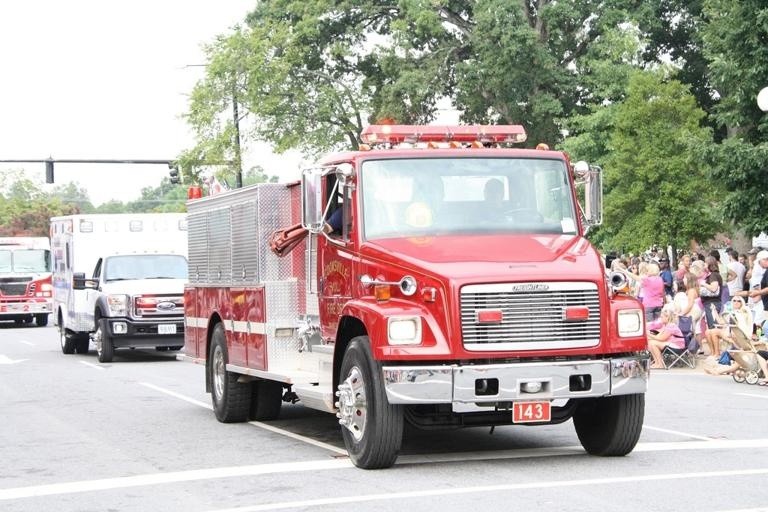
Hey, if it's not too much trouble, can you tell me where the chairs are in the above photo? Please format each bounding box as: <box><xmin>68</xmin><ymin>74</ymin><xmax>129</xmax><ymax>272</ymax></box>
<box><xmin>648</xmin><ymin>300</ymin><xmax>766</xmax><ymax>369</ymax></box>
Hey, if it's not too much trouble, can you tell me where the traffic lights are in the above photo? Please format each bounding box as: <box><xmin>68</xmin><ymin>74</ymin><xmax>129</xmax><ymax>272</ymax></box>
<box><xmin>168</xmin><ymin>163</ymin><xmax>184</xmax><ymax>184</ymax></box>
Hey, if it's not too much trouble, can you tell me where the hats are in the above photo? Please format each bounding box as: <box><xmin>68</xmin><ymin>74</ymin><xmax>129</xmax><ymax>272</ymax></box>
<box><xmin>726</xmin><ymin>247</ymin><xmax>733</xmax><ymax>252</ymax></box>
<box><xmin>747</xmin><ymin>249</ymin><xmax>757</xmax><ymax>254</ymax></box>
<box><xmin>658</xmin><ymin>258</ymin><xmax>669</xmax><ymax>261</ymax></box>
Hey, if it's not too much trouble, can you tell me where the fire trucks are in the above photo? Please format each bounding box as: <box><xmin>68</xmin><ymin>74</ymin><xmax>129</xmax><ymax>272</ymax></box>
<box><xmin>0</xmin><ymin>235</ymin><xmax>53</xmax><ymax>326</ymax></box>
<box><xmin>174</xmin><ymin>120</ymin><xmax>651</xmax><ymax>469</ymax></box>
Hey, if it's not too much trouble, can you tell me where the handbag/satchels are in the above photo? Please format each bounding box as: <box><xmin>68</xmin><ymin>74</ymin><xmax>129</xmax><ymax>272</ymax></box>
<box><xmin>688</xmin><ymin>336</ymin><xmax>700</xmax><ymax>353</ymax></box>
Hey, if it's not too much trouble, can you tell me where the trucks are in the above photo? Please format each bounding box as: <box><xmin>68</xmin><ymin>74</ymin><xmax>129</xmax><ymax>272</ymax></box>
<box><xmin>47</xmin><ymin>212</ymin><xmax>189</xmax><ymax>364</ymax></box>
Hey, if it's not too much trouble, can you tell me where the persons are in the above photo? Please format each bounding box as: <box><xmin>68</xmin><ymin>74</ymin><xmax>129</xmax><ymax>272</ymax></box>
<box><xmin>416</xmin><ymin>173</ymin><xmax>447</xmax><ymax>204</ymax></box>
<box><xmin>181</xmin><ymin>289</ymin><xmax>265</xmax><ymax>351</ymax></box>
<box><xmin>609</xmin><ymin>242</ymin><xmax>767</xmax><ymax>388</ymax></box>
<box><xmin>321</xmin><ymin>206</ymin><xmax>344</xmax><ymax>238</ymax></box>
<box><xmin>471</xmin><ymin>177</ymin><xmax>525</xmax><ymax>229</ymax></box>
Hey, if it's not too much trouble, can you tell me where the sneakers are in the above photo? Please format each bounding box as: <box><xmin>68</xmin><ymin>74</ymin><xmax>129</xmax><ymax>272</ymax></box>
<box><xmin>707</xmin><ymin>354</ymin><xmax>721</xmax><ymax>361</ymax></box>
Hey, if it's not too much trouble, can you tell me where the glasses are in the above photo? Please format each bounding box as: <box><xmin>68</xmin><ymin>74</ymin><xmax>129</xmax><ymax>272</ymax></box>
<box><xmin>732</xmin><ymin>299</ymin><xmax>741</xmax><ymax>302</ymax></box>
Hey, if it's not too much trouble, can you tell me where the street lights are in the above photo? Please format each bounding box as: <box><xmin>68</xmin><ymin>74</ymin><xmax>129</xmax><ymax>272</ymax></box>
<box><xmin>173</xmin><ymin>61</ymin><xmax>242</xmax><ymax>186</ymax></box>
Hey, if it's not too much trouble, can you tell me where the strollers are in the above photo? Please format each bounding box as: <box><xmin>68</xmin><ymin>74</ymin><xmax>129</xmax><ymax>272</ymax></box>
<box><xmin>720</xmin><ymin>311</ymin><xmax>768</xmax><ymax>384</ymax></box>
